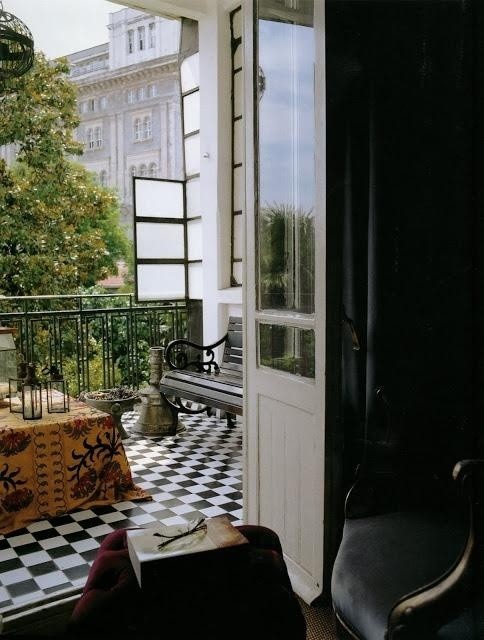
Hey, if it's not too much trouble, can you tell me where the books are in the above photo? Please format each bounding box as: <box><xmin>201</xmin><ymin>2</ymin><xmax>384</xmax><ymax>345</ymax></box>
<box><xmin>125</xmin><ymin>515</ymin><xmax>251</xmax><ymax>590</ymax></box>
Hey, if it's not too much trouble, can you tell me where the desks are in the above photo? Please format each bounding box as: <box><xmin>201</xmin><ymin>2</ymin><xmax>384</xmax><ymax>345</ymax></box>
<box><xmin>0</xmin><ymin>386</ymin><xmax>114</xmax><ymax>528</ymax></box>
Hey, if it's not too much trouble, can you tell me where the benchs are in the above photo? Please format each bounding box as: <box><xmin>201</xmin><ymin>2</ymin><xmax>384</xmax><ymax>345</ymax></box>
<box><xmin>157</xmin><ymin>315</ymin><xmax>244</xmax><ymax>434</ymax></box>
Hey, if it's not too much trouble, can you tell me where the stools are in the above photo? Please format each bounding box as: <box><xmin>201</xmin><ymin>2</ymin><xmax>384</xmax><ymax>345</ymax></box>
<box><xmin>67</xmin><ymin>525</ymin><xmax>307</xmax><ymax>640</ymax></box>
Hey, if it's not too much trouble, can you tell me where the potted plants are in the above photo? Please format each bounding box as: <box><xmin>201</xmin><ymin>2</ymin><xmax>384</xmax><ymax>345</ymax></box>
<box><xmin>84</xmin><ymin>388</ymin><xmax>143</xmax><ymax>440</ymax></box>
